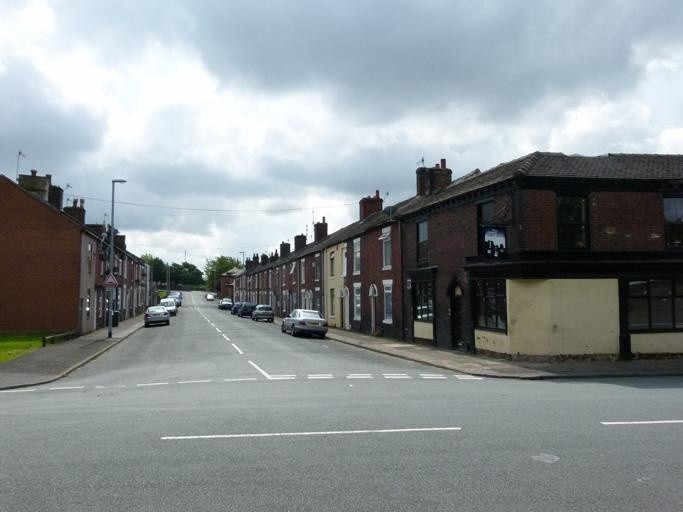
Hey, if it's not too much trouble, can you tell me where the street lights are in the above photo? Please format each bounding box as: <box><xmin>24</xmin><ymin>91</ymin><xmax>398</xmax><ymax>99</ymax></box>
<box><xmin>238</xmin><ymin>251</ymin><xmax>245</xmax><ymax>270</ymax></box>
<box><xmin>107</xmin><ymin>177</ymin><xmax>129</xmax><ymax>338</ymax></box>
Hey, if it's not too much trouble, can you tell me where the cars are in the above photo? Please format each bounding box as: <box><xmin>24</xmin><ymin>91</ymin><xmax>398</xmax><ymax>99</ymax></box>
<box><xmin>143</xmin><ymin>289</ymin><xmax>183</xmax><ymax>327</ymax></box>
<box><xmin>218</xmin><ymin>297</ymin><xmax>276</xmax><ymax>323</ymax></box>
<box><xmin>281</xmin><ymin>308</ymin><xmax>330</xmax><ymax>339</ymax></box>
<box><xmin>415</xmin><ymin>304</ymin><xmax>432</xmax><ymax>322</ymax></box>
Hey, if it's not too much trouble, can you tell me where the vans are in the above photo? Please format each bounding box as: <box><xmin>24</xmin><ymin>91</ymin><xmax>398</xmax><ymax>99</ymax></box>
<box><xmin>204</xmin><ymin>293</ymin><xmax>215</xmax><ymax>301</ymax></box>
